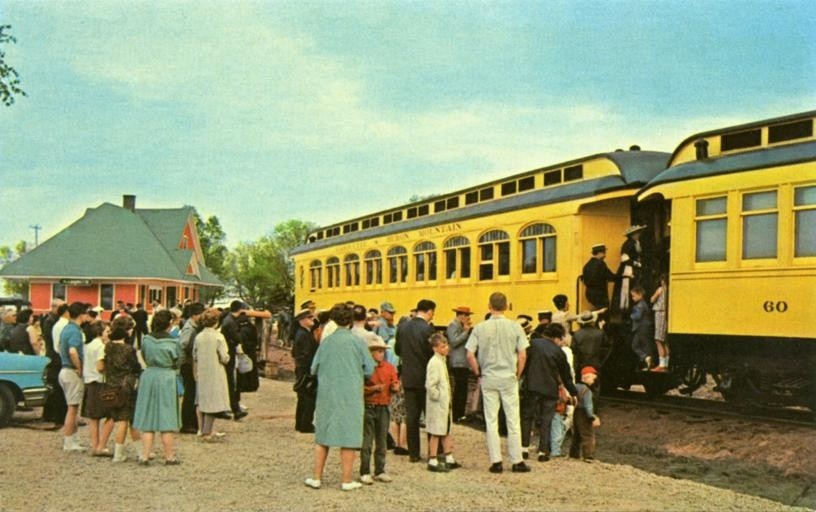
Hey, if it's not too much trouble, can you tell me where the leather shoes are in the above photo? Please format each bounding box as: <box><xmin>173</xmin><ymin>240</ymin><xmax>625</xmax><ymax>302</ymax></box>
<box><xmin>489</xmin><ymin>465</ymin><xmax>503</xmax><ymax>472</ymax></box>
<box><xmin>512</xmin><ymin>464</ymin><xmax>532</xmax><ymax>472</ymax></box>
<box><xmin>427</xmin><ymin>462</ymin><xmax>450</xmax><ymax>473</ymax></box>
<box><xmin>445</xmin><ymin>460</ymin><xmax>463</xmax><ymax>470</ymax></box>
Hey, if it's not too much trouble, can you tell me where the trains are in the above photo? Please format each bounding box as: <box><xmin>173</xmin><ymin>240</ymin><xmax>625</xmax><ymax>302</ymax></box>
<box><xmin>287</xmin><ymin>103</ymin><xmax>815</xmax><ymax>430</ymax></box>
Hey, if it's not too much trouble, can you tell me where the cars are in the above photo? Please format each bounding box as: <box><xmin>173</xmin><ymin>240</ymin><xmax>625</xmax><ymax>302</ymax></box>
<box><xmin>0</xmin><ymin>346</ymin><xmax>54</xmax><ymax>430</ymax></box>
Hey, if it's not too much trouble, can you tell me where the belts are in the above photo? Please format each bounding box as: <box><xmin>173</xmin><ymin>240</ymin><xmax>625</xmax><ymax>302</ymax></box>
<box><xmin>61</xmin><ymin>364</ymin><xmax>77</xmax><ymax>370</ymax></box>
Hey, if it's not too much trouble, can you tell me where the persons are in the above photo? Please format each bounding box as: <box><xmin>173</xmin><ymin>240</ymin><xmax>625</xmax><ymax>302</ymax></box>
<box><xmin>580</xmin><ymin>244</ymin><xmax>617</xmax><ymax>321</ymax></box>
<box><xmin>278</xmin><ymin>292</ymin><xmax>612</xmax><ymax>489</ymax></box>
<box><xmin>609</xmin><ymin>225</ymin><xmax>669</xmax><ymax>372</ymax></box>
<box><xmin>1</xmin><ymin>300</ymin><xmax>257</xmax><ymax>465</ymax></box>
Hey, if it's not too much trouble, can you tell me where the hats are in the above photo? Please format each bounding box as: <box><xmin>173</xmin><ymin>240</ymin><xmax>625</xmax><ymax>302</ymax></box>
<box><xmin>380</xmin><ymin>301</ymin><xmax>396</xmax><ymax>314</ymax></box>
<box><xmin>590</xmin><ymin>244</ymin><xmax>608</xmax><ymax>253</ymax></box>
<box><xmin>294</xmin><ymin>309</ymin><xmax>314</xmax><ymax>322</ymax></box>
<box><xmin>516</xmin><ymin>318</ymin><xmax>532</xmax><ymax>329</ymax></box>
<box><xmin>452</xmin><ymin>306</ymin><xmax>474</xmax><ymax>316</ymax></box>
<box><xmin>300</xmin><ymin>299</ymin><xmax>316</xmax><ymax>310</ymax></box>
<box><xmin>365</xmin><ymin>335</ymin><xmax>392</xmax><ymax>350</ymax></box>
<box><xmin>622</xmin><ymin>223</ymin><xmax>649</xmax><ymax>236</ymax></box>
<box><xmin>537</xmin><ymin>310</ymin><xmax>553</xmax><ymax>320</ymax></box>
<box><xmin>581</xmin><ymin>365</ymin><xmax>599</xmax><ymax>375</ymax></box>
<box><xmin>183</xmin><ymin>305</ymin><xmax>204</xmax><ymax>319</ymax></box>
<box><xmin>575</xmin><ymin>309</ymin><xmax>599</xmax><ymax>324</ymax></box>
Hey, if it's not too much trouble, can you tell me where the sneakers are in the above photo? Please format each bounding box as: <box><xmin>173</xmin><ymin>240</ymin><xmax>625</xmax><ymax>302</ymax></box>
<box><xmin>522</xmin><ymin>452</ymin><xmax>528</xmax><ymax>460</ymax></box>
<box><xmin>393</xmin><ymin>446</ymin><xmax>410</xmax><ymax>455</ymax></box>
<box><xmin>373</xmin><ymin>472</ymin><xmax>392</xmax><ymax>483</ymax></box>
<box><xmin>359</xmin><ymin>474</ymin><xmax>374</xmax><ymax>484</ymax></box>
<box><xmin>641</xmin><ymin>356</ymin><xmax>652</xmax><ymax>371</ymax></box>
<box><xmin>234</xmin><ymin>411</ymin><xmax>248</xmax><ymax>422</ymax></box>
<box><xmin>341</xmin><ymin>481</ymin><xmax>363</xmax><ymax>491</ymax></box>
<box><xmin>538</xmin><ymin>455</ymin><xmax>550</xmax><ymax>462</ymax></box>
<box><xmin>62</xmin><ymin>418</ymin><xmax>226</xmax><ymax>466</ymax></box>
<box><xmin>303</xmin><ymin>478</ymin><xmax>322</xmax><ymax>489</ymax></box>
<box><xmin>215</xmin><ymin>411</ymin><xmax>232</xmax><ymax>420</ymax></box>
<box><xmin>650</xmin><ymin>366</ymin><xmax>669</xmax><ymax>373</ymax></box>
<box><xmin>584</xmin><ymin>457</ymin><xmax>601</xmax><ymax>465</ymax></box>
<box><xmin>409</xmin><ymin>454</ymin><xmax>421</xmax><ymax>462</ymax></box>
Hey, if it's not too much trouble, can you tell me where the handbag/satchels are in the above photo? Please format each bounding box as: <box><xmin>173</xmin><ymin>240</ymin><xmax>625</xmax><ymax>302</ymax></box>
<box><xmin>292</xmin><ymin>363</ymin><xmax>320</xmax><ymax>399</ymax></box>
<box><xmin>236</xmin><ymin>351</ymin><xmax>254</xmax><ymax>376</ymax></box>
<box><xmin>98</xmin><ymin>384</ymin><xmax>128</xmax><ymax>411</ymax></box>
